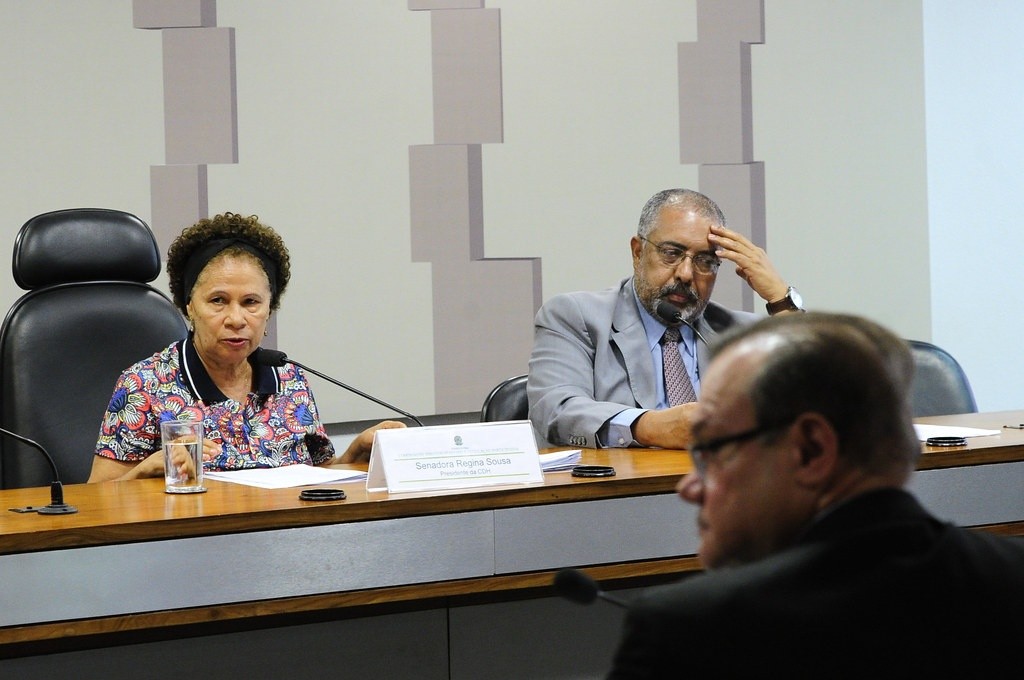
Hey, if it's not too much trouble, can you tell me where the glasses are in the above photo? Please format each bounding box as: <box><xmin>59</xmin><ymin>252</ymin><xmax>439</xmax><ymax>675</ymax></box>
<box><xmin>642</xmin><ymin>237</ymin><xmax>721</xmax><ymax>273</ymax></box>
<box><xmin>689</xmin><ymin>430</ymin><xmax>756</xmax><ymax>472</ymax></box>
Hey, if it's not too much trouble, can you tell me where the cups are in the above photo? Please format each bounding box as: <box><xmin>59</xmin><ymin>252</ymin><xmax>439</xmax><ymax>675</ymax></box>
<box><xmin>160</xmin><ymin>420</ymin><xmax>205</xmax><ymax>494</ymax></box>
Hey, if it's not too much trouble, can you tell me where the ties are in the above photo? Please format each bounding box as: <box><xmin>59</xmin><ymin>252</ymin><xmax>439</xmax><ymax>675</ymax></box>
<box><xmin>661</xmin><ymin>328</ymin><xmax>697</xmax><ymax>408</ymax></box>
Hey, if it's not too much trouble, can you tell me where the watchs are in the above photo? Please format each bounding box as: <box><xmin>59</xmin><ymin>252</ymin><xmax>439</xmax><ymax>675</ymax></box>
<box><xmin>766</xmin><ymin>287</ymin><xmax>803</xmax><ymax>315</ymax></box>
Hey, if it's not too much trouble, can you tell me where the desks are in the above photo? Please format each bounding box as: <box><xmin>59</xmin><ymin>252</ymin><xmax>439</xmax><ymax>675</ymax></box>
<box><xmin>0</xmin><ymin>412</ymin><xmax>1024</xmax><ymax>680</ymax></box>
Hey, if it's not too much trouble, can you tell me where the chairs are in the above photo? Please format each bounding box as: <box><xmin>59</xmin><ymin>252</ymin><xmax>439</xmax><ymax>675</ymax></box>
<box><xmin>896</xmin><ymin>341</ymin><xmax>978</xmax><ymax>417</ymax></box>
<box><xmin>482</xmin><ymin>375</ymin><xmax>529</xmax><ymax>424</ymax></box>
<box><xmin>0</xmin><ymin>206</ymin><xmax>190</xmax><ymax>490</ymax></box>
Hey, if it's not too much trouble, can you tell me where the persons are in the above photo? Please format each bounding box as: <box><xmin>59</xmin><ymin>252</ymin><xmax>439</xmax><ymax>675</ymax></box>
<box><xmin>525</xmin><ymin>189</ymin><xmax>806</xmax><ymax>451</ymax></box>
<box><xmin>87</xmin><ymin>212</ymin><xmax>409</xmax><ymax>483</ymax></box>
<box><xmin>609</xmin><ymin>312</ymin><xmax>1024</xmax><ymax>680</ymax></box>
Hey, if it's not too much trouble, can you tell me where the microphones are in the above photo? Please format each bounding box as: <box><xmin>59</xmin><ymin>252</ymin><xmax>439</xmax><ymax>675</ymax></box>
<box><xmin>554</xmin><ymin>569</ymin><xmax>630</xmax><ymax>613</ymax></box>
<box><xmin>255</xmin><ymin>347</ymin><xmax>425</xmax><ymax>428</ymax></box>
<box><xmin>657</xmin><ymin>302</ymin><xmax>709</xmax><ymax>347</ymax></box>
